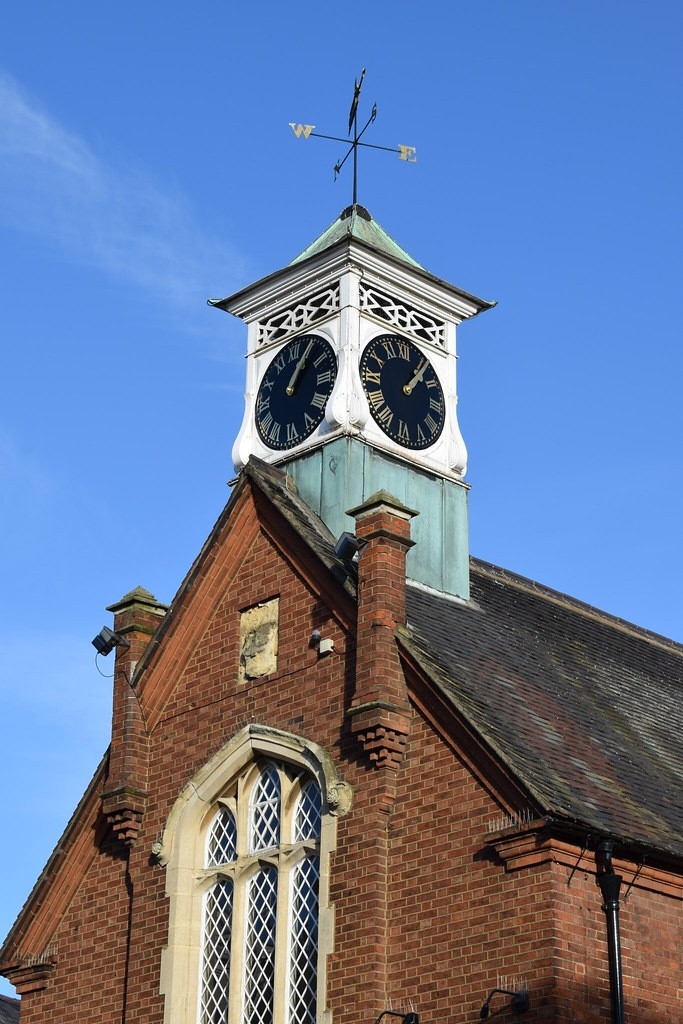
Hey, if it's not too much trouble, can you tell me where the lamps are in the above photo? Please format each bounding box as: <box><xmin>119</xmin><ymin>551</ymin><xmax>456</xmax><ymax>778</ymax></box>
<box><xmin>93</xmin><ymin>626</ymin><xmax>130</xmax><ymax>657</ymax></box>
<box><xmin>334</xmin><ymin>532</ymin><xmax>370</xmax><ymax>561</ymax></box>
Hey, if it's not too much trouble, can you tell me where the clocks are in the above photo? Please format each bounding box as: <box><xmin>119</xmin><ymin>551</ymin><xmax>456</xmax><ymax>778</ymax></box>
<box><xmin>359</xmin><ymin>333</ymin><xmax>446</xmax><ymax>450</ymax></box>
<box><xmin>254</xmin><ymin>334</ymin><xmax>338</xmax><ymax>451</ymax></box>
